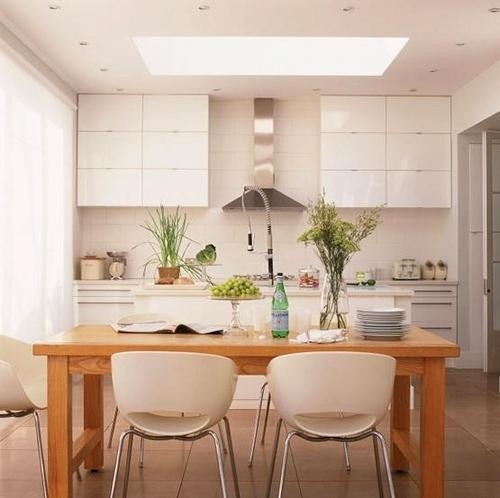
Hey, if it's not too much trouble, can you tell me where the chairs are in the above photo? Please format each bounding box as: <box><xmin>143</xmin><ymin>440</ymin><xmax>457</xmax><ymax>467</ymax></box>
<box><xmin>260</xmin><ymin>343</ymin><xmax>403</xmax><ymax>497</ymax></box>
<box><xmin>0</xmin><ymin>332</ymin><xmax>84</xmax><ymax>498</ymax></box>
<box><xmin>101</xmin><ymin>343</ymin><xmax>246</xmax><ymax>497</ymax></box>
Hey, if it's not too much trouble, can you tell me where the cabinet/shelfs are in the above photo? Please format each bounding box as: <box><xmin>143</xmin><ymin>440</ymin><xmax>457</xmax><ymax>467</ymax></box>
<box><xmin>389</xmin><ymin>280</ymin><xmax>459</xmax><ymax>346</ymax></box>
<box><xmin>316</xmin><ymin>91</ymin><xmax>453</xmax><ymax>211</ymax></box>
<box><xmin>75</xmin><ymin>90</ymin><xmax>213</xmax><ymax>210</ymax></box>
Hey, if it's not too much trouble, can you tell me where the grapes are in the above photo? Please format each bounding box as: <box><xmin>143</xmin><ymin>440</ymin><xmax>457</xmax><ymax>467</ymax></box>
<box><xmin>210</xmin><ymin>276</ymin><xmax>259</xmax><ymax>297</ymax></box>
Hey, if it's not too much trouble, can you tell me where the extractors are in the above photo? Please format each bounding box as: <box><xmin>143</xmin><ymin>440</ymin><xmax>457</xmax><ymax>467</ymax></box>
<box><xmin>220</xmin><ymin>98</ymin><xmax>307</xmax><ymax>214</ymax></box>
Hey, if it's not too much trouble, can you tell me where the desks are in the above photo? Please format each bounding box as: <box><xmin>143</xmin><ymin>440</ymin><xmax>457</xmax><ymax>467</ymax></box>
<box><xmin>30</xmin><ymin>324</ymin><xmax>458</xmax><ymax>498</ymax></box>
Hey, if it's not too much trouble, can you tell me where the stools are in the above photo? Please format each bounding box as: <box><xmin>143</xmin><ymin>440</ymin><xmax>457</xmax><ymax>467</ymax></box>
<box><xmin>103</xmin><ymin>403</ymin><xmax>227</xmax><ymax>472</ymax></box>
<box><xmin>247</xmin><ymin>381</ymin><xmax>354</xmax><ymax>474</ymax></box>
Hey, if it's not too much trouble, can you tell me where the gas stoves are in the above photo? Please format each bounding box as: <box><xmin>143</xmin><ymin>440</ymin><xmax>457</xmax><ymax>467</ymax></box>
<box><xmin>234</xmin><ymin>273</ymin><xmax>299</xmax><ymax>286</ymax></box>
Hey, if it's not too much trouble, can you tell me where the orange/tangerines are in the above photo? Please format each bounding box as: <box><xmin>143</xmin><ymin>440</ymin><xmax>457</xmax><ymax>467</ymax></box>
<box><xmin>367</xmin><ymin>279</ymin><xmax>376</xmax><ymax>286</ymax></box>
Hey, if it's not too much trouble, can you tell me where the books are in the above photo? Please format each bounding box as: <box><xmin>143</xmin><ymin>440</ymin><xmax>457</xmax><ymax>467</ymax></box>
<box><xmin>119</xmin><ymin>319</ymin><xmax>226</xmax><ymax>335</ymax></box>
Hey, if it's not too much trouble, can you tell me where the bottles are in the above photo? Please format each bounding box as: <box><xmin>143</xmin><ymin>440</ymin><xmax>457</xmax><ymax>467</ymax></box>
<box><xmin>297</xmin><ymin>265</ymin><xmax>320</xmax><ymax>289</ymax></box>
<box><xmin>419</xmin><ymin>261</ymin><xmax>447</xmax><ymax>281</ymax></box>
<box><xmin>271</xmin><ymin>271</ymin><xmax>289</xmax><ymax>339</ymax></box>
<box><xmin>356</xmin><ymin>265</ymin><xmax>377</xmax><ymax>282</ymax></box>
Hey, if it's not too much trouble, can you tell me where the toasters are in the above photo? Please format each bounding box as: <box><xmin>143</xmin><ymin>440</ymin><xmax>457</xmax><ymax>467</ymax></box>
<box><xmin>392</xmin><ymin>258</ymin><xmax>421</xmax><ymax>279</ymax></box>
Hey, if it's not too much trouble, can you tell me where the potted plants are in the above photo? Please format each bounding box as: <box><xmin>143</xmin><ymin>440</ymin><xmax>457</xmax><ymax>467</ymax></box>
<box><xmin>295</xmin><ymin>183</ymin><xmax>386</xmax><ymax>330</ymax></box>
<box><xmin>124</xmin><ymin>198</ymin><xmax>217</xmax><ymax>289</ymax></box>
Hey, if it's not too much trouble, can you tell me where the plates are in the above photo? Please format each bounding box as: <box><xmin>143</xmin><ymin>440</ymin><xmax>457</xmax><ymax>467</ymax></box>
<box><xmin>354</xmin><ymin>306</ymin><xmax>409</xmax><ymax>340</ymax></box>
